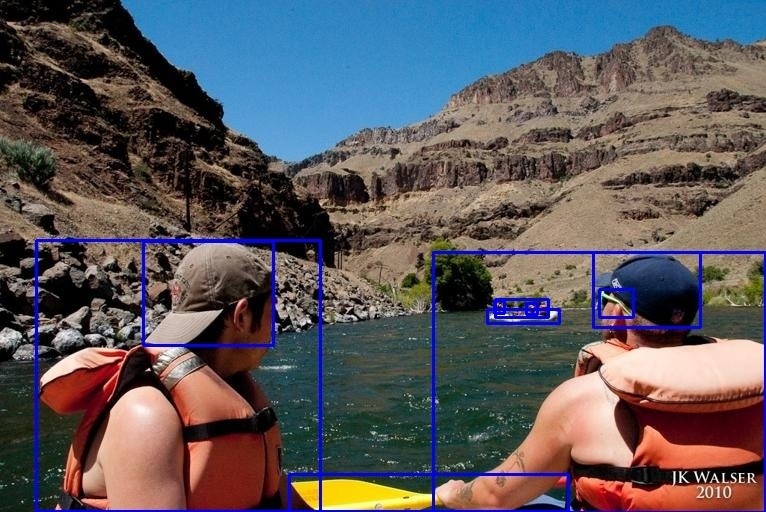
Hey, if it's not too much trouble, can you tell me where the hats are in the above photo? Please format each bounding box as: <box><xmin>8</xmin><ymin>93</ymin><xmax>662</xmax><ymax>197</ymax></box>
<box><xmin>144</xmin><ymin>242</ymin><xmax>274</xmax><ymax>346</ymax></box>
<box><xmin>593</xmin><ymin>252</ymin><xmax>701</xmax><ymax>328</ymax></box>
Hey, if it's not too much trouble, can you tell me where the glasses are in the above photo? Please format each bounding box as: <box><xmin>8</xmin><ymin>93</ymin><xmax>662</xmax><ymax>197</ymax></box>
<box><xmin>600</xmin><ymin>289</ymin><xmax>634</xmax><ymax>317</ymax></box>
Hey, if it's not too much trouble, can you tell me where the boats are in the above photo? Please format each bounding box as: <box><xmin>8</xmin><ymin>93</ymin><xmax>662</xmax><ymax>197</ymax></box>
<box><xmin>488</xmin><ymin>309</ymin><xmax>559</xmax><ymax>323</ymax></box>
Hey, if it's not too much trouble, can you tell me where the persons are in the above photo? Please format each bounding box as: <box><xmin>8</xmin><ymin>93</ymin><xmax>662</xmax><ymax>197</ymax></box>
<box><xmin>434</xmin><ymin>253</ymin><xmax>766</xmax><ymax>512</ymax></box>
<box><xmin>495</xmin><ymin>299</ymin><xmax>548</xmax><ymax>317</ymax></box>
<box><xmin>36</xmin><ymin>241</ymin><xmax>320</xmax><ymax>512</ymax></box>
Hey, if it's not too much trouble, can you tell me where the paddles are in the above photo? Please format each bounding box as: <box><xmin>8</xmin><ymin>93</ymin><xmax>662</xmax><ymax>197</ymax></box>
<box><xmin>290</xmin><ymin>475</ymin><xmax>568</xmax><ymax>512</ymax></box>
